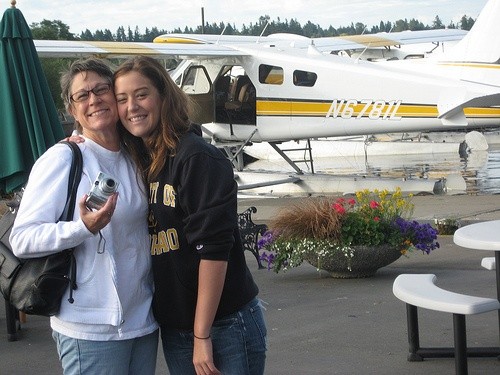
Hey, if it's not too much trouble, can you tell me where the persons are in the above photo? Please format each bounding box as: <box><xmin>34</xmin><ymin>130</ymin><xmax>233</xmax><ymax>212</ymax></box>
<box><xmin>58</xmin><ymin>54</ymin><xmax>267</xmax><ymax>375</ymax></box>
<box><xmin>7</xmin><ymin>57</ymin><xmax>162</xmax><ymax>375</ymax></box>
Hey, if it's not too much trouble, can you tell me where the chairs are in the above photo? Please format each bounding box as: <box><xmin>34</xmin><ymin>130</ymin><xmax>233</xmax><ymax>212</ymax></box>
<box><xmin>225</xmin><ymin>75</ymin><xmax>251</xmax><ymax>110</ymax></box>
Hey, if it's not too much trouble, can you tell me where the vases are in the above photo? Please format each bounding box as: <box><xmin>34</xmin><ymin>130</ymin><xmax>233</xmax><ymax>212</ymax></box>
<box><xmin>303</xmin><ymin>245</ymin><xmax>402</xmax><ymax>279</ymax></box>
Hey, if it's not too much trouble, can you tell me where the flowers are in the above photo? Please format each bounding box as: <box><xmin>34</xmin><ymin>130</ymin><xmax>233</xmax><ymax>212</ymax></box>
<box><xmin>257</xmin><ymin>186</ymin><xmax>440</xmax><ymax>274</ymax></box>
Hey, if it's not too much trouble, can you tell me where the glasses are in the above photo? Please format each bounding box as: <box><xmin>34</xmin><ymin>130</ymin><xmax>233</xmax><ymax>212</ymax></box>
<box><xmin>70</xmin><ymin>82</ymin><xmax>111</xmax><ymax>102</ymax></box>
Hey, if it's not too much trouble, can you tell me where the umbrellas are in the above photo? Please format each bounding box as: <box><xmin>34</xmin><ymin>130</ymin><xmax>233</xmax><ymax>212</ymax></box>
<box><xmin>0</xmin><ymin>0</ymin><xmax>67</xmax><ymax>196</ymax></box>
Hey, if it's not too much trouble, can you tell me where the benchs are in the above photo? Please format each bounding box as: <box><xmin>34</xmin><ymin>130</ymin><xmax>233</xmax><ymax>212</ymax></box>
<box><xmin>480</xmin><ymin>257</ymin><xmax>496</xmax><ymax>271</ymax></box>
<box><xmin>236</xmin><ymin>206</ymin><xmax>268</xmax><ymax>271</ymax></box>
<box><xmin>393</xmin><ymin>274</ymin><xmax>500</xmax><ymax>375</ymax></box>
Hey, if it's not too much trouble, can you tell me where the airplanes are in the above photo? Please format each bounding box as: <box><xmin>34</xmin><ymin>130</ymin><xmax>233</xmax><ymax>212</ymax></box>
<box><xmin>33</xmin><ymin>0</ymin><xmax>500</xmax><ymax>142</ymax></box>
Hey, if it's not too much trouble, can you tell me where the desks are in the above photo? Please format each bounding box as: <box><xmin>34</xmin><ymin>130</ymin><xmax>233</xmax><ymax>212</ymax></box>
<box><xmin>453</xmin><ymin>219</ymin><xmax>500</xmax><ymax>358</ymax></box>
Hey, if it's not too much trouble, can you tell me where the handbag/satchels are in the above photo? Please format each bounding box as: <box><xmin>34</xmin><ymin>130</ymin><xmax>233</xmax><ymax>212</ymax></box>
<box><xmin>0</xmin><ymin>141</ymin><xmax>84</xmax><ymax>316</ymax></box>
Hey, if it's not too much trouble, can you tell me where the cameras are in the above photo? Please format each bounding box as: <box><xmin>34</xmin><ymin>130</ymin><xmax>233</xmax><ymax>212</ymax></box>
<box><xmin>85</xmin><ymin>171</ymin><xmax>120</xmax><ymax>211</ymax></box>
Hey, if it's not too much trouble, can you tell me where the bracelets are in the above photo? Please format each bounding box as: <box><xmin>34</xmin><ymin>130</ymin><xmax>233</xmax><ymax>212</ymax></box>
<box><xmin>193</xmin><ymin>333</ymin><xmax>210</xmax><ymax>339</ymax></box>
